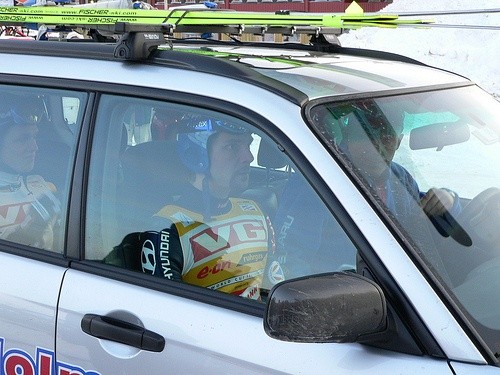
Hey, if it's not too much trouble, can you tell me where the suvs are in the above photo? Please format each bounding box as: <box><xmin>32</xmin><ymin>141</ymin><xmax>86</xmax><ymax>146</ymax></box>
<box><xmin>0</xmin><ymin>22</ymin><xmax>500</xmax><ymax>374</ymax></box>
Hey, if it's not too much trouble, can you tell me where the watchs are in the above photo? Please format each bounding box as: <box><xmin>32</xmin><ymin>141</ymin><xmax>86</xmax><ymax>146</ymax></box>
<box><xmin>449</xmin><ymin>190</ymin><xmax>456</xmax><ymax>200</ymax></box>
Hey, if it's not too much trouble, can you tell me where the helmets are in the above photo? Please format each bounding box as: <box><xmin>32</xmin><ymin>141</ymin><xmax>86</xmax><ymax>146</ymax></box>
<box><xmin>0</xmin><ymin>90</ymin><xmax>51</xmax><ymax>127</ymax></box>
<box><xmin>176</xmin><ymin>111</ymin><xmax>252</xmax><ymax>173</ymax></box>
<box><xmin>150</xmin><ymin>109</ymin><xmax>184</xmax><ymax>141</ymax></box>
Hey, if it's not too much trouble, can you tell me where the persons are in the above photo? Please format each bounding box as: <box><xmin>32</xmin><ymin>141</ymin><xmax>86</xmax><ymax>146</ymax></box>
<box><xmin>267</xmin><ymin>99</ymin><xmax>463</xmax><ymax>284</ymax></box>
<box><xmin>66</xmin><ymin>27</ymin><xmax>86</xmax><ymax>39</ymax></box>
<box><xmin>0</xmin><ymin>92</ymin><xmax>66</xmax><ymax>253</ymax></box>
<box><xmin>137</xmin><ymin>113</ymin><xmax>287</xmax><ymax>304</ymax></box>
